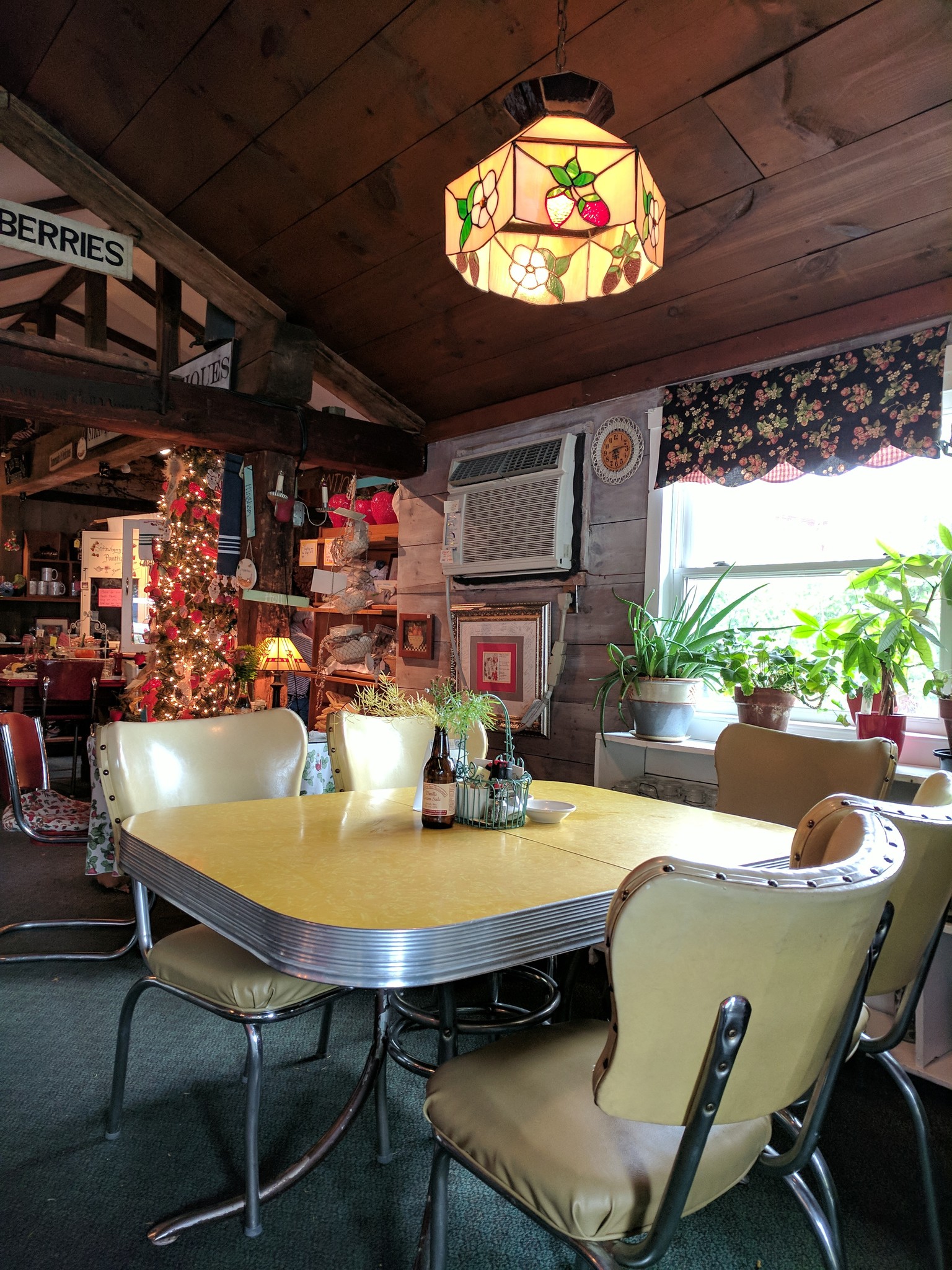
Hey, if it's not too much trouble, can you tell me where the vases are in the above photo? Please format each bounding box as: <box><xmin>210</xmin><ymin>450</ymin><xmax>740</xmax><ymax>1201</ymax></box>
<box><xmin>232</xmin><ymin>679</ymin><xmax>252</xmax><ymax>715</ymax></box>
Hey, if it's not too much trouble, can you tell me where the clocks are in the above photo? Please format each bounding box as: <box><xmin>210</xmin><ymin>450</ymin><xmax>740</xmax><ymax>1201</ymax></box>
<box><xmin>590</xmin><ymin>415</ymin><xmax>645</xmax><ymax>487</ymax></box>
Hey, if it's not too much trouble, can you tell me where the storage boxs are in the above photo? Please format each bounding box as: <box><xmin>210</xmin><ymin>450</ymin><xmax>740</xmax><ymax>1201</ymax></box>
<box><xmin>370</xmin><ymin>624</ymin><xmax>396</xmax><ymax>657</ymax></box>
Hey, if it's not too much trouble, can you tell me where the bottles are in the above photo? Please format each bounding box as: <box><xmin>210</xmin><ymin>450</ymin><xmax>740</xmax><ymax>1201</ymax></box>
<box><xmin>100</xmin><ymin>634</ymin><xmax>109</xmax><ymax>659</ymax></box>
<box><xmin>422</xmin><ymin>726</ymin><xmax>456</xmax><ymax>829</ymax></box>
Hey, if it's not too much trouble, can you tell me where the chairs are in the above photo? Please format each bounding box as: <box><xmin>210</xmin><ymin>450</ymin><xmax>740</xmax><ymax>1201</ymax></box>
<box><xmin>94</xmin><ymin>708</ymin><xmax>952</xmax><ymax>1270</ymax></box>
<box><xmin>32</xmin><ymin>657</ymin><xmax>106</xmax><ymax>799</ymax></box>
<box><xmin>0</xmin><ymin>710</ymin><xmax>156</xmax><ymax>966</ymax></box>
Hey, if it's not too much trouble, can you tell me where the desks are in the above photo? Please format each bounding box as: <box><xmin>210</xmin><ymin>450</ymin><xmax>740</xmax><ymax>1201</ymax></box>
<box><xmin>116</xmin><ymin>779</ymin><xmax>798</xmax><ymax>1250</ymax></box>
<box><xmin>0</xmin><ymin>670</ymin><xmax>128</xmax><ymax>744</ymax></box>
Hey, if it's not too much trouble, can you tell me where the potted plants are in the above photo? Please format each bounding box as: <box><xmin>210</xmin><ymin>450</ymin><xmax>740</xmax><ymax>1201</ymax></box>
<box><xmin>789</xmin><ymin>567</ymin><xmax>929</xmax><ymax>728</ymax></box>
<box><xmin>922</xmin><ymin>668</ymin><xmax>952</xmax><ymax>754</ymax></box>
<box><xmin>587</xmin><ymin>560</ymin><xmax>804</xmax><ymax>744</ymax></box>
<box><xmin>836</xmin><ymin>521</ymin><xmax>952</xmax><ymax>764</ymax></box>
<box><xmin>698</xmin><ymin>624</ymin><xmax>860</xmax><ymax>734</ymax></box>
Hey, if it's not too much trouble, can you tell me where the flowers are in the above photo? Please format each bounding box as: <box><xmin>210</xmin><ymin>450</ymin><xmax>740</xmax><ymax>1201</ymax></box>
<box><xmin>3</xmin><ymin>531</ymin><xmax>21</xmax><ymax>552</ymax></box>
<box><xmin>210</xmin><ymin>635</ymin><xmax>276</xmax><ymax>695</ymax></box>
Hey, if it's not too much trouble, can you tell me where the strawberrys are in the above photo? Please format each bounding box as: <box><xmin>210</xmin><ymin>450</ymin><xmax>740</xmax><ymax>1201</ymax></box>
<box><xmin>327</xmin><ymin>481</ymin><xmax>400</xmax><ymax>527</ymax></box>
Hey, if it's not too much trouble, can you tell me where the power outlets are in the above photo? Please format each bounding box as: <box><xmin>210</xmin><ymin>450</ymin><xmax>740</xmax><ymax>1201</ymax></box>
<box><xmin>562</xmin><ymin>585</ymin><xmax>579</xmax><ymax>616</ymax></box>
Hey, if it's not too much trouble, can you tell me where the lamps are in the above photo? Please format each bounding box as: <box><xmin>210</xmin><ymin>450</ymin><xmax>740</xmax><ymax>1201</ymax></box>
<box><xmin>266</xmin><ymin>467</ymin><xmax>336</xmax><ymax>527</ymax></box>
<box><xmin>443</xmin><ymin>0</ymin><xmax>667</xmax><ymax>307</ymax></box>
<box><xmin>253</xmin><ymin>627</ymin><xmax>312</xmax><ymax>709</ymax></box>
<box><xmin>121</xmin><ymin>463</ymin><xmax>131</xmax><ymax>473</ymax></box>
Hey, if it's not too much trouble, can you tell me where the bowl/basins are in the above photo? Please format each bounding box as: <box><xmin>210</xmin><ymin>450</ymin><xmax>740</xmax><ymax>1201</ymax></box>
<box><xmin>525</xmin><ymin>800</ymin><xmax>576</xmax><ymax>824</ymax></box>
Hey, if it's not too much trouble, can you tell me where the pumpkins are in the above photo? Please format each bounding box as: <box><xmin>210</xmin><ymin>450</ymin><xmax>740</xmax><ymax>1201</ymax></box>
<box><xmin>74</xmin><ymin>643</ymin><xmax>95</xmax><ymax>658</ymax></box>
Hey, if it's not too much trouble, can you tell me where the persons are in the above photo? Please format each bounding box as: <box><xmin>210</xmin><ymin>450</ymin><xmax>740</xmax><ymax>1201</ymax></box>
<box><xmin>287</xmin><ymin>606</ymin><xmax>316</xmax><ymax>731</ymax></box>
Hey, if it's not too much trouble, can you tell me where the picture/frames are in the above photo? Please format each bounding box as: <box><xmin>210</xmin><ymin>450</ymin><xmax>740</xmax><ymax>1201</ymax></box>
<box><xmin>447</xmin><ymin>600</ymin><xmax>553</xmax><ymax>741</ymax></box>
<box><xmin>33</xmin><ymin>616</ymin><xmax>70</xmax><ymax>638</ymax></box>
<box><xmin>385</xmin><ymin>553</ymin><xmax>398</xmax><ymax>582</ymax></box>
<box><xmin>398</xmin><ymin>612</ymin><xmax>434</xmax><ymax>660</ymax></box>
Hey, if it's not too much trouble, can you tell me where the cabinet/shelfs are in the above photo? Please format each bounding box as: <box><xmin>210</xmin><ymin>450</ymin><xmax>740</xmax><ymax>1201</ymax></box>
<box><xmin>0</xmin><ymin>531</ymin><xmax>82</xmax><ymax>604</ymax></box>
<box><xmin>292</xmin><ymin>523</ymin><xmax>399</xmax><ymax>737</ymax></box>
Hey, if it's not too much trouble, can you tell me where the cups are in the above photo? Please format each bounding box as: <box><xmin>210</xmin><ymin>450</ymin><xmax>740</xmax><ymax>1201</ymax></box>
<box><xmin>29</xmin><ymin>625</ymin><xmax>39</xmax><ymax>636</ymax></box>
<box><xmin>507</xmin><ymin>795</ymin><xmax>523</xmax><ymax>821</ymax></box>
<box><xmin>68</xmin><ymin>580</ymin><xmax>97</xmax><ymax>596</ymax></box>
<box><xmin>29</xmin><ymin>567</ymin><xmax>66</xmax><ymax>596</ymax></box>
<box><xmin>455</xmin><ymin>783</ymin><xmax>490</xmax><ymax>819</ymax></box>
